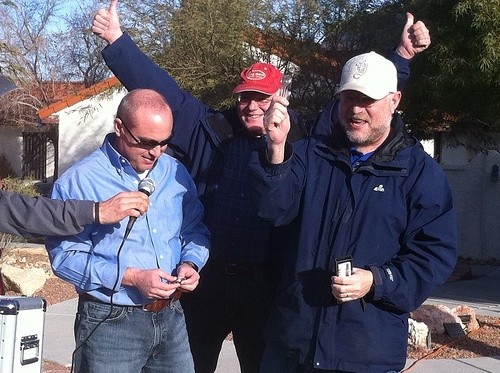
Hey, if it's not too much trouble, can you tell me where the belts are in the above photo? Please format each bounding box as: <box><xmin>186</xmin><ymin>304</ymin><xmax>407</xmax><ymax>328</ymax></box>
<box><xmin>84</xmin><ymin>293</ymin><xmax>171</xmax><ymax>311</ymax></box>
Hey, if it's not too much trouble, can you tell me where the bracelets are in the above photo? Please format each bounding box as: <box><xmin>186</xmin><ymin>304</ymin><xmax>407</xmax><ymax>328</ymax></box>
<box><xmin>95</xmin><ymin>202</ymin><xmax>100</xmax><ymax>225</ymax></box>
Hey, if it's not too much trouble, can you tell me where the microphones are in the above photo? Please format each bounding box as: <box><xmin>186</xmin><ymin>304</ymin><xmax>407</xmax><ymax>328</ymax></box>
<box><xmin>123</xmin><ymin>179</ymin><xmax>156</xmax><ymax>239</ymax></box>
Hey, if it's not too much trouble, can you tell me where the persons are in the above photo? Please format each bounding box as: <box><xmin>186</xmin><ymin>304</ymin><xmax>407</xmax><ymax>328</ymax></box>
<box><xmin>46</xmin><ymin>89</ymin><xmax>210</xmax><ymax>373</ymax></box>
<box><xmin>0</xmin><ymin>189</ymin><xmax>150</xmax><ymax>241</ymax></box>
<box><xmin>91</xmin><ymin>0</ymin><xmax>430</xmax><ymax>373</ymax></box>
<box><xmin>244</xmin><ymin>52</ymin><xmax>458</xmax><ymax>373</ymax></box>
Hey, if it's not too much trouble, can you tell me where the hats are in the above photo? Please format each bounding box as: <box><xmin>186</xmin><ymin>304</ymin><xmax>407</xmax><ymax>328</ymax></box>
<box><xmin>334</xmin><ymin>50</ymin><xmax>397</xmax><ymax>100</ymax></box>
<box><xmin>233</xmin><ymin>62</ymin><xmax>283</xmax><ymax>95</ymax></box>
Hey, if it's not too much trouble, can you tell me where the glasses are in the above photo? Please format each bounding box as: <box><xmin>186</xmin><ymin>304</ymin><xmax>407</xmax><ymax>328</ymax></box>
<box><xmin>237</xmin><ymin>96</ymin><xmax>271</xmax><ymax>105</ymax></box>
<box><xmin>116</xmin><ymin>115</ymin><xmax>171</xmax><ymax>148</ymax></box>
